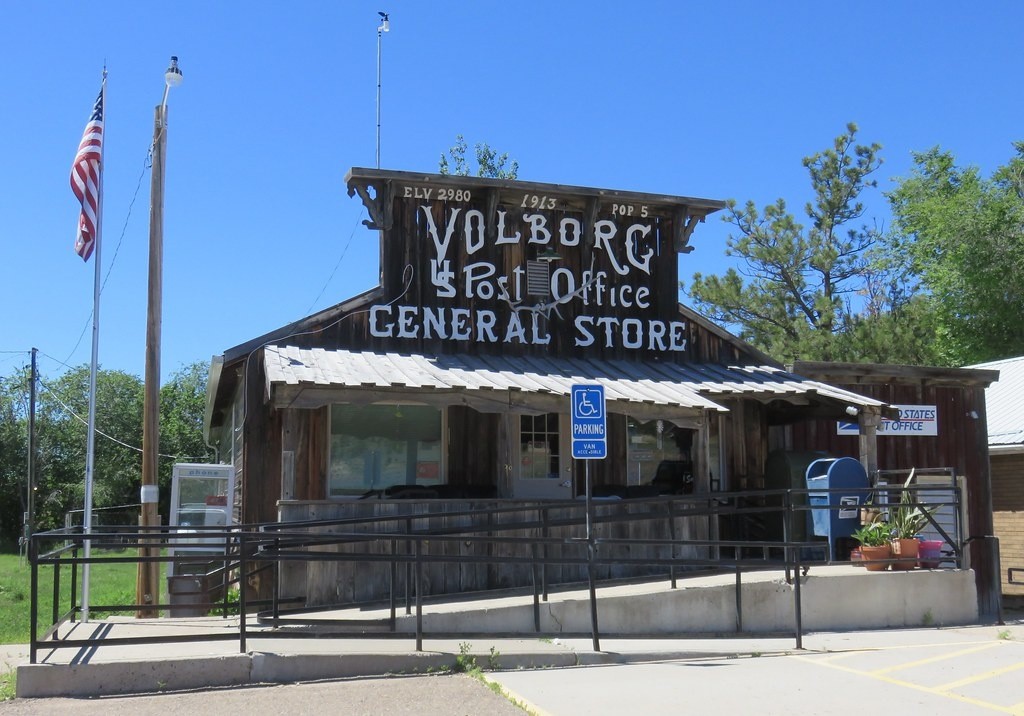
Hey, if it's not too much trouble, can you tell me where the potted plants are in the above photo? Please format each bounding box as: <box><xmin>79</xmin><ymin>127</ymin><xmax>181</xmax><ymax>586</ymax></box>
<box><xmin>849</xmin><ymin>485</ymin><xmax>945</xmax><ymax>572</ymax></box>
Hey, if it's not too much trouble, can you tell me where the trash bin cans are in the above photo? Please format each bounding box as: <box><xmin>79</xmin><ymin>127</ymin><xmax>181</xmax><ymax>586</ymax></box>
<box><xmin>804</xmin><ymin>457</ymin><xmax>870</xmax><ymax>561</ymax></box>
<box><xmin>166</xmin><ymin>574</ymin><xmax>206</xmax><ymax>618</ymax></box>
